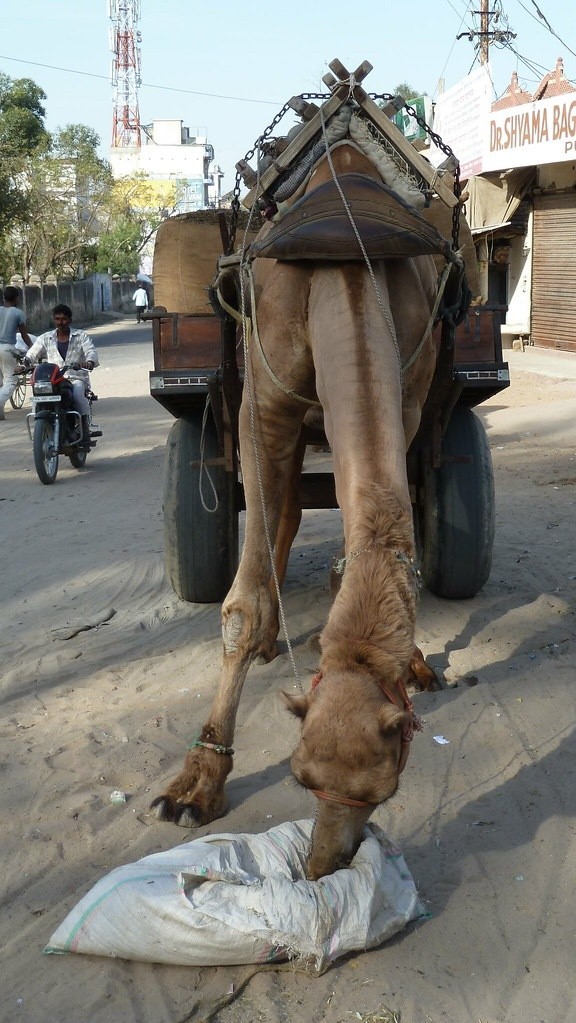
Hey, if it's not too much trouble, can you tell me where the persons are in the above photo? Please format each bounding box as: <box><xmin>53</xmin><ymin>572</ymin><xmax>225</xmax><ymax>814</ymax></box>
<box><xmin>14</xmin><ymin>304</ymin><xmax>100</xmax><ymax>449</ymax></box>
<box><xmin>133</xmin><ymin>285</ymin><xmax>148</xmax><ymax>324</ymax></box>
<box><xmin>142</xmin><ymin>283</ymin><xmax>150</xmax><ymax>305</ymax></box>
<box><xmin>0</xmin><ymin>286</ymin><xmax>33</xmax><ymax>420</ymax></box>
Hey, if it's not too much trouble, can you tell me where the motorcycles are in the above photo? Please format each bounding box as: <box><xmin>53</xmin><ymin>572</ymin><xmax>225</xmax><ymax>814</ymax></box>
<box><xmin>11</xmin><ymin>358</ymin><xmax>104</xmax><ymax>485</ymax></box>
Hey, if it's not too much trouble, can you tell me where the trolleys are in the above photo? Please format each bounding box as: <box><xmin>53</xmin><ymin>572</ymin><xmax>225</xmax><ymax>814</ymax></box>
<box><xmin>9</xmin><ymin>350</ymin><xmax>42</xmax><ymax>411</ymax></box>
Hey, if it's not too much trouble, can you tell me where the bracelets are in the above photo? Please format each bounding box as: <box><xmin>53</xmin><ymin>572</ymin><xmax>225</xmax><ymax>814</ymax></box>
<box><xmin>20</xmin><ymin>365</ymin><xmax>26</xmax><ymax>368</ymax></box>
<box><xmin>88</xmin><ymin>362</ymin><xmax>92</xmax><ymax>365</ymax></box>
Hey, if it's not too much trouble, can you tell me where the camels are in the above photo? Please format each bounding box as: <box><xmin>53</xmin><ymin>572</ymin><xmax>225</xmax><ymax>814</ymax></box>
<box><xmin>142</xmin><ymin>122</ymin><xmax>465</xmax><ymax>880</ymax></box>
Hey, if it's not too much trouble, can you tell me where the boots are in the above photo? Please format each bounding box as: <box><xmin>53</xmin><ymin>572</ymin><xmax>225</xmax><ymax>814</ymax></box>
<box><xmin>80</xmin><ymin>415</ymin><xmax>90</xmax><ymax>441</ymax></box>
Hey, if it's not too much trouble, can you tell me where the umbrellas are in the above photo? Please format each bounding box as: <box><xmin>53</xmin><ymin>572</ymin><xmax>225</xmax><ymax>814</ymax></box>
<box><xmin>137</xmin><ymin>274</ymin><xmax>152</xmax><ymax>285</ymax></box>
